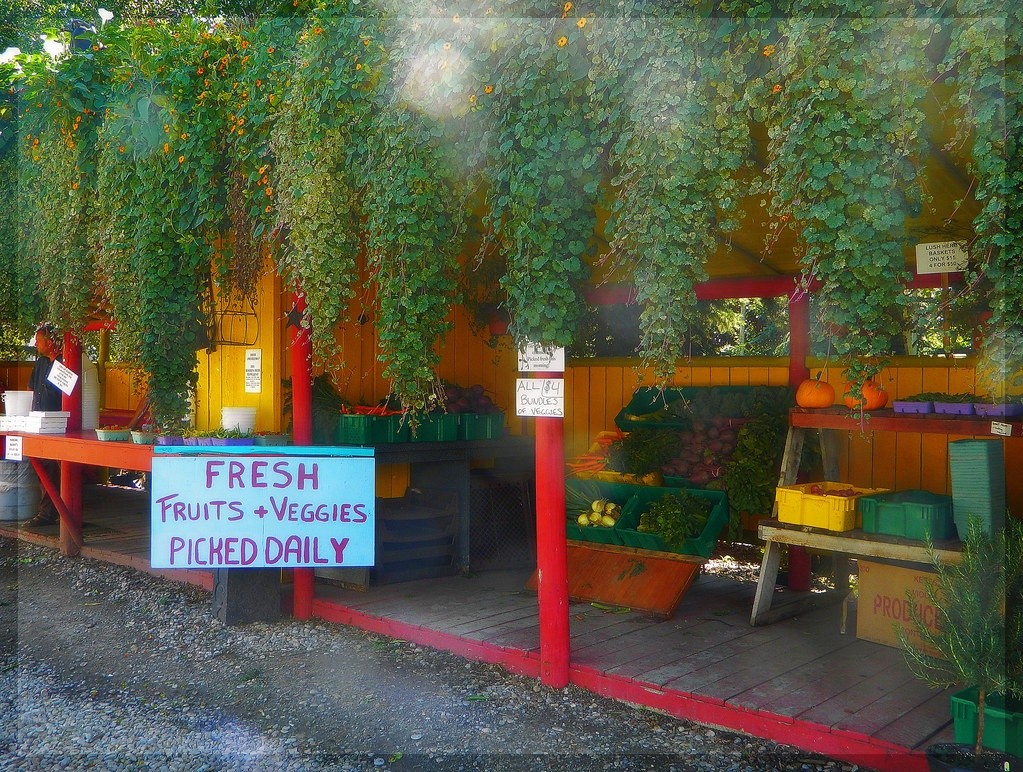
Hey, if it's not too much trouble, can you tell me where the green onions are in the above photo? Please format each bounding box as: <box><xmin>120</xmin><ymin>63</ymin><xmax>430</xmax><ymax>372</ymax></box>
<box><xmin>627</xmin><ymin>399</ymin><xmax>686</xmax><ymax>423</ymax></box>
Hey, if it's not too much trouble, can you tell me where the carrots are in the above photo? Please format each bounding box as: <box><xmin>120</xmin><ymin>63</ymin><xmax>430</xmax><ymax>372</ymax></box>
<box><xmin>566</xmin><ymin>423</ymin><xmax>622</xmax><ymax>476</ymax></box>
<box><xmin>339</xmin><ymin>405</ymin><xmax>406</xmax><ymax>415</ymax></box>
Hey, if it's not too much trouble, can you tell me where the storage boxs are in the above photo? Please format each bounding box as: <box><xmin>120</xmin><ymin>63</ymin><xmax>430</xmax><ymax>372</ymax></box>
<box><xmin>565</xmin><ymin>382</ymin><xmax>1023</xmax><ymax>558</ymax></box>
<box><xmin>857</xmin><ymin>558</ymin><xmax>997</xmax><ymax>663</ymax></box>
<box><xmin>950</xmin><ymin>682</ymin><xmax>1023</xmax><ymax>758</ymax></box>
<box><xmin>94</xmin><ymin>425</ymin><xmax>292</xmax><ymax>445</ymax></box>
<box><xmin>336</xmin><ymin>405</ymin><xmax>508</xmax><ymax>446</ymax></box>
<box><xmin>25</xmin><ymin>411</ymin><xmax>71</xmax><ymax>434</ymax></box>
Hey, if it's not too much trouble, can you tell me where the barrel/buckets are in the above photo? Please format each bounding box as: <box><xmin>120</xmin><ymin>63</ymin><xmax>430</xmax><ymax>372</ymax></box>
<box><xmin>1</xmin><ymin>391</ymin><xmax>33</xmax><ymax>416</ymax></box>
<box><xmin>221</xmin><ymin>408</ymin><xmax>255</xmax><ymax>436</ymax></box>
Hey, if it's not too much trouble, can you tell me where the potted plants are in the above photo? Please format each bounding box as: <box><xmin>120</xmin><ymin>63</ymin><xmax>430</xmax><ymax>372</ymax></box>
<box><xmin>896</xmin><ymin>509</ymin><xmax>1023</xmax><ymax>772</ymax></box>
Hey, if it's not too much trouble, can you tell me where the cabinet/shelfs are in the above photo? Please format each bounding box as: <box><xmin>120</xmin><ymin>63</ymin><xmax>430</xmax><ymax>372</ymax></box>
<box><xmin>749</xmin><ymin>410</ymin><xmax>1016</xmax><ymax>632</ymax></box>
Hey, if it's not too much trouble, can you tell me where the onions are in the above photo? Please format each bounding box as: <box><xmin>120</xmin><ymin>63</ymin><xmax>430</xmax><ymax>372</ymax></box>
<box><xmin>577</xmin><ymin>499</ymin><xmax>617</xmax><ymax>528</ymax></box>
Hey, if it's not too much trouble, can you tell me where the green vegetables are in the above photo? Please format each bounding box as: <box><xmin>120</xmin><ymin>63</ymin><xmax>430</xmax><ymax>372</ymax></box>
<box><xmin>636</xmin><ymin>486</ymin><xmax>716</xmax><ymax>549</ymax></box>
<box><xmin>721</xmin><ymin>411</ymin><xmax>823</xmax><ymax>541</ymax></box>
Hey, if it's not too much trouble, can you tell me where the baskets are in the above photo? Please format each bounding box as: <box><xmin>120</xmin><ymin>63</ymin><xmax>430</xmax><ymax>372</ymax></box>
<box><xmin>891</xmin><ymin>397</ymin><xmax>1022</xmax><ymax>417</ymax></box>
<box><xmin>94</xmin><ymin>425</ymin><xmax>288</xmax><ymax>446</ymax></box>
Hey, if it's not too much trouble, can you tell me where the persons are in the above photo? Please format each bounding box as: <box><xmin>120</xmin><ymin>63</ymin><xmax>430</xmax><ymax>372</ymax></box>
<box><xmin>20</xmin><ymin>326</ymin><xmax>63</xmax><ymax>527</ymax></box>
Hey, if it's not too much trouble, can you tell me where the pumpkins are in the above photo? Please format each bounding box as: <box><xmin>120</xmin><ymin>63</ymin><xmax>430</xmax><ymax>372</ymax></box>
<box><xmin>796</xmin><ymin>371</ymin><xmax>834</xmax><ymax>408</ymax></box>
<box><xmin>844</xmin><ymin>375</ymin><xmax>888</xmax><ymax>411</ymax></box>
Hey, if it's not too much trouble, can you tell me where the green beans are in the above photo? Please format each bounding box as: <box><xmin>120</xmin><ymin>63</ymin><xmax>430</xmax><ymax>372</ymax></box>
<box><xmin>183</xmin><ymin>423</ymin><xmax>252</xmax><ymax>439</ymax></box>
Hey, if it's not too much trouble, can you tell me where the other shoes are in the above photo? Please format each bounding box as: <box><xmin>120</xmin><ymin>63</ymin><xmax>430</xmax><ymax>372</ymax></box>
<box><xmin>21</xmin><ymin>514</ymin><xmax>60</xmax><ymax>526</ymax></box>
